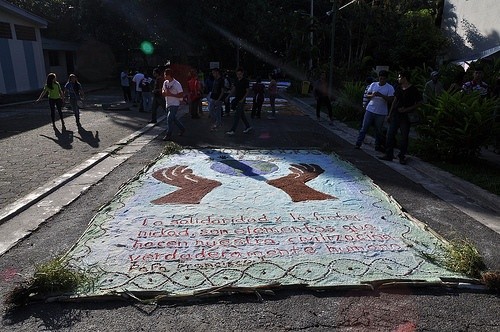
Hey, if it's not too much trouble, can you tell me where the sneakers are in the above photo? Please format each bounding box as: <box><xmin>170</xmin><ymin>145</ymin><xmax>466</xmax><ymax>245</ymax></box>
<box><xmin>354</xmin><ymin>145</ymin><xmax>360</xmax><ymax>149</ymax></box>
<box><xmin>400</xmin><ymin>159</ymin><xmax>406</xmax><ymax>165</ymax></box>
<box><xmin>162</xmin><ymin>136</ymin><xmax>173</xmax><ymax>141</ymax></box>
<box><xmin>267</xmin><ymin>115</ymin><xmax>276</xmax><ymax>120</ymax></box>
<box><xmin>317</xmin><ymin>117</ymin><xmax>321</xmax><ymax>121</ymax></box>
<box><xmin>147</xmin><ymin>119</ymin><xmax>157</xmax><ymax>123</ymax></box>
<box><xmin>178</xmin><ymin>128</ymin><xmax>186</xmax><ymax>136</ymax></box>
<box><xmin>375</xmin><ymin>144</ymin><xmax>385</xmax><ymax>153</ymax></box>
<box><xmin>378</xmin><ymin>155</ymin><xmax>393</xmax><ymax>161</ymax></box>
<box><xmin>225</xmin><ymin>131</ymin><xmax>235</xmax><ymax>135</ymax></box>
<box><xmin>330</xmin><ymin>116</ymin><xmax>336</xmax><ymax>121</ymax></box>
<box><xmin>243</xmin><ymin>127</ymin><xmax>252</xmax><ymax>133</ymax></box>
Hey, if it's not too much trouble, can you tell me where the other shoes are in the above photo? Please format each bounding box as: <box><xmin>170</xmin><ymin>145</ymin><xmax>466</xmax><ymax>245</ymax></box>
<box><xmin>53</xmin><ymin>124</ymin><xmax>56</xmax><ymax>129</ymax></box>
<box><xmin>62</xmin><ymin>124</ymin><xmax>66</xmax><ymax>129</ymax></box>
<box><xmin>76</xmin><ymin>119</ymin><xmax>80</xmax><ymax>126</ymax></box>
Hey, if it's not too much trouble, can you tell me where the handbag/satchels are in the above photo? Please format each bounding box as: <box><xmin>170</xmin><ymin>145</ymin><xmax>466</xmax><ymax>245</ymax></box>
<box><xmin>77</xmin><ymin>98</ymin><xmax>87</xmax><ymax>108</ymax></box>
<box><xmin>192</xmin><ymin>80</ymin><xmax>204</xmax><ymax>102</ymax></box>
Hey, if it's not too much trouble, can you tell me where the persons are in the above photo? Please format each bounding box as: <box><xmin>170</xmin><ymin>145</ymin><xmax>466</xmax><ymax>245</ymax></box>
<box><xmin>33</xmin><ymin>72</ymin><xmax>65</xmax><ymax>126</ymax></box>
<box><xmin>459</xmin><ymin>66</ymin><xmax>489</xmax><ymax>105</ymax></box>
<box><xmin>354</xmin><ymin>69</ymin><xmax>421</xmax><ymax>164</ymax></box>
<box><xmin>422</xmin><ymin>71</ymin><xmax>443</xmax><ymax>105</ymax></box>
<box><xmin>64</xmin><ymin>73</ymin><xmax>85</xmax><ymax>129</ymax></box>
<box><xmin>119</xmin><ymin>65</ymin><xmax>278</xmax><ymax>140</ymax></box>
<box><xmin>312</xmin><ymin>71</ymin><xmax>337</xmax><ymax>123</ymax></box>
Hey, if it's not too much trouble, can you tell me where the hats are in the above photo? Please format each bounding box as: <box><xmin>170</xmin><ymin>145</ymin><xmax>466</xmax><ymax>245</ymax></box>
<box><xmin>431</xmin><ymin>71</ymin><xmax>439</xmax><ymax>76</ymax></box>
